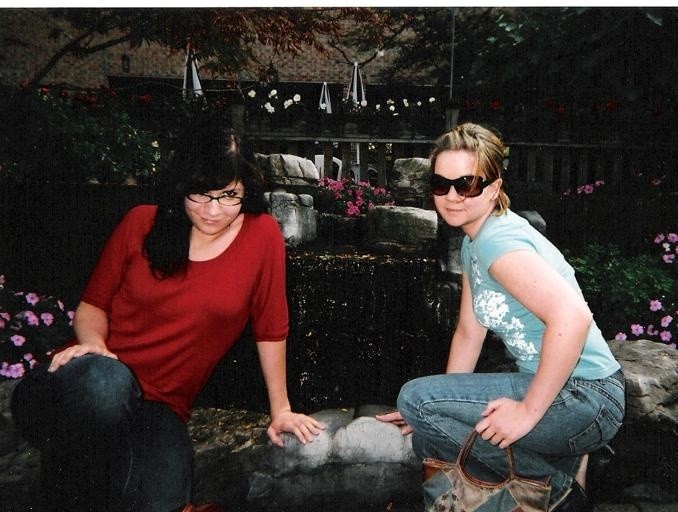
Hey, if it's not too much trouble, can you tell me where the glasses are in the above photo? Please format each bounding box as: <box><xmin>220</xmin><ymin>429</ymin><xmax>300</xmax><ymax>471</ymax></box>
<box><xmin>429</xmin><ymin>173</ymin><xmax>497</xmax><ymax>197</ymax></box>
<box><xmin>186</xmin><ymin>190</ymin><xmax>249</xmax><ymax>206</ymax></box>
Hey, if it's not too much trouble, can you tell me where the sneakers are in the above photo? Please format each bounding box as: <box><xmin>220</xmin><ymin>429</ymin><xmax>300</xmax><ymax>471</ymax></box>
<box><xmin>551</xmin><ymin>479</ymin><xmax>593</xmax><ymax>511</ymax></box>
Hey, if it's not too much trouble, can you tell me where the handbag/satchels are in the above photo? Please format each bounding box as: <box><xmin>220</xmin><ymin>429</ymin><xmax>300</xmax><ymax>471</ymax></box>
<box><xmin>422</xmin><ymin>458</ymin><xmax>552</xmax><ymax>512</ymax></box>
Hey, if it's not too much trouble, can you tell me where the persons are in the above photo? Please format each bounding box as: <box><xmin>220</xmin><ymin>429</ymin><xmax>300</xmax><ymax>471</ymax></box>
<box><xmin>11</xmin><ymin>128</ymin><xmax>324</xmax><ymax>512</ymax></box>
<box><xmin>376</xmin><ymin>123</ymin><xmax>625</xmax><ymax>512</ymax></box>
